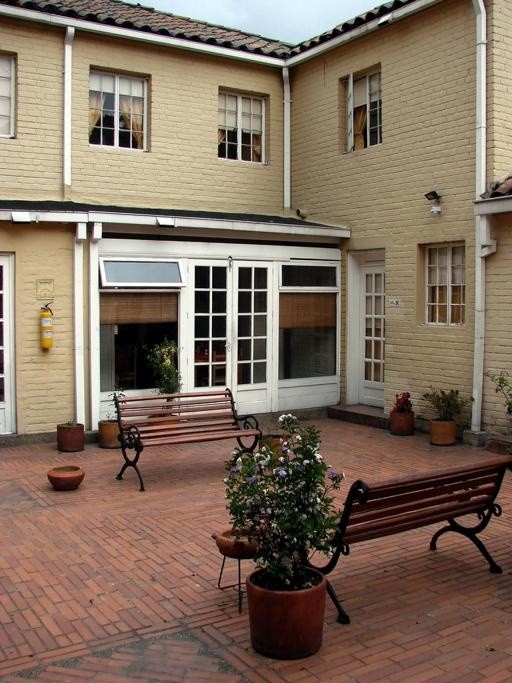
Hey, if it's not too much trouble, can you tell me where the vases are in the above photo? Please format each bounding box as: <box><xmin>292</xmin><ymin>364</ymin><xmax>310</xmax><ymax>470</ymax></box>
<box><xmin>388</xmin><ymin>410</ymin><xmax>414</xmax><ymax>436</ymax></box>
<box><xmin>45</xmin><ymin>464</ymin><xmax>84</xmax><ymax>490</ymax></box>
<box><xmin>246</xmin><ymin>567</ymin><xmax>328</xmax><ymax>660</ymax></box>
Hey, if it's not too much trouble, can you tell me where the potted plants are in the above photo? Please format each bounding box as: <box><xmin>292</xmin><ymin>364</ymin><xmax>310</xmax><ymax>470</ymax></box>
<box><xmin>140</xmin><ymin>338</ymin><xmax>183</xmax><ymax>438</ymax></box>
<box><xmin>423</xmin><ymin>384</ymin><xmax>474</xmax><ymax>445</ymax></box>
<box><xmin>97</xmin><ymin>412</ymin><xmax>125</xmax><ymax>448</ymax></box>
<box><xmin>56</xmin><ymin>415</ymin><xmax>83</xmax><ymax>451</ymax></box>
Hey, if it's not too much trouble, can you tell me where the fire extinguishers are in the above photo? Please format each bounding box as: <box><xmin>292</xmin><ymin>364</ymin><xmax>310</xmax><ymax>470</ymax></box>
<box><xmin>40</xmin><ymin>301</ymin><xmax>54</xmax><ymax>349</ymax></box>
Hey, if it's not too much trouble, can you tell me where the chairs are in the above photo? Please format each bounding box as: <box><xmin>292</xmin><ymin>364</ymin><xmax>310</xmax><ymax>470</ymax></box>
<box><xmin>200</xmin><ymin>346</ymin><xmax>245</xmax><ymax>387</ymax></box>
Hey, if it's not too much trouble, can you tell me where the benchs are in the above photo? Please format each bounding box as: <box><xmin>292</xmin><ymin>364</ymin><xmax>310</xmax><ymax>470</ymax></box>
<box><xmin>212</xmin><ymin>452</ymin><xmax>512</xmax><ymax>624</ymax></box>
<box><xmin>107</xmin><ymin>387</ymin><xmax>267</xmax><ymax>493</ymax></box>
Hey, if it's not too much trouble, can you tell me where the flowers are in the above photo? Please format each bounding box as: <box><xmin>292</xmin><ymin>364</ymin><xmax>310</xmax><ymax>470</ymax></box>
<box><xmin>390</xmin><ymin>391</ymin><xmax>411</xmax><ymax>412</ymax></box>
<box><xmin>225</xmin><ymin>416</ymin><xmax>346</xmax><ymax>590</ymax></box>
